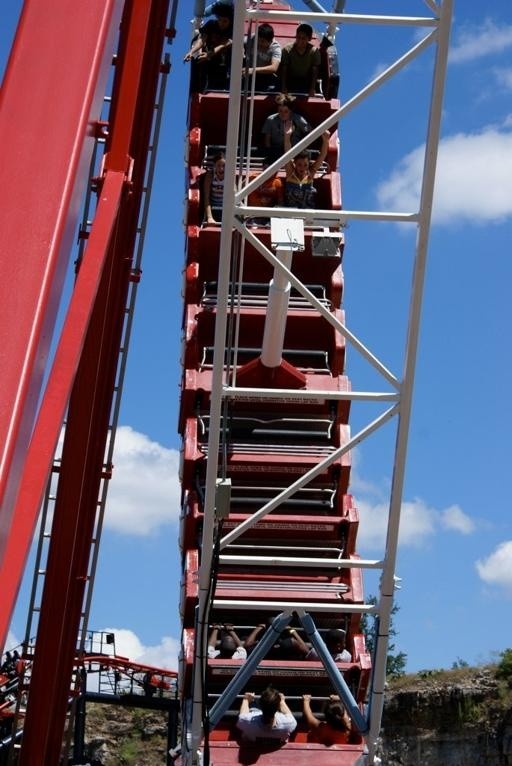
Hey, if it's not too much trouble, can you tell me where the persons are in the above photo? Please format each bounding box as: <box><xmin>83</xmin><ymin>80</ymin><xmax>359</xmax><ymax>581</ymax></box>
<box><xmin>208</xmin><ymin>621</ymin><xmax>352</xmax><ymax>745</ymax></box>
<box><xmin>0</xmin><ymin>649</ymin><xmax>158</xmax><ymax>740</ymax></box>
<box><xmin>183</xmin><ymin>4</ymin><xmax>331</xmax><ymax>225</ymax></box>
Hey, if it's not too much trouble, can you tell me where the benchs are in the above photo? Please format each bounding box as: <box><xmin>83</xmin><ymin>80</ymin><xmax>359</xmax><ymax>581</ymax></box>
<box><xmin>180</xmin><ymin>0</ymin><xmax>373</xmax><ymax>765</ymax></box>
